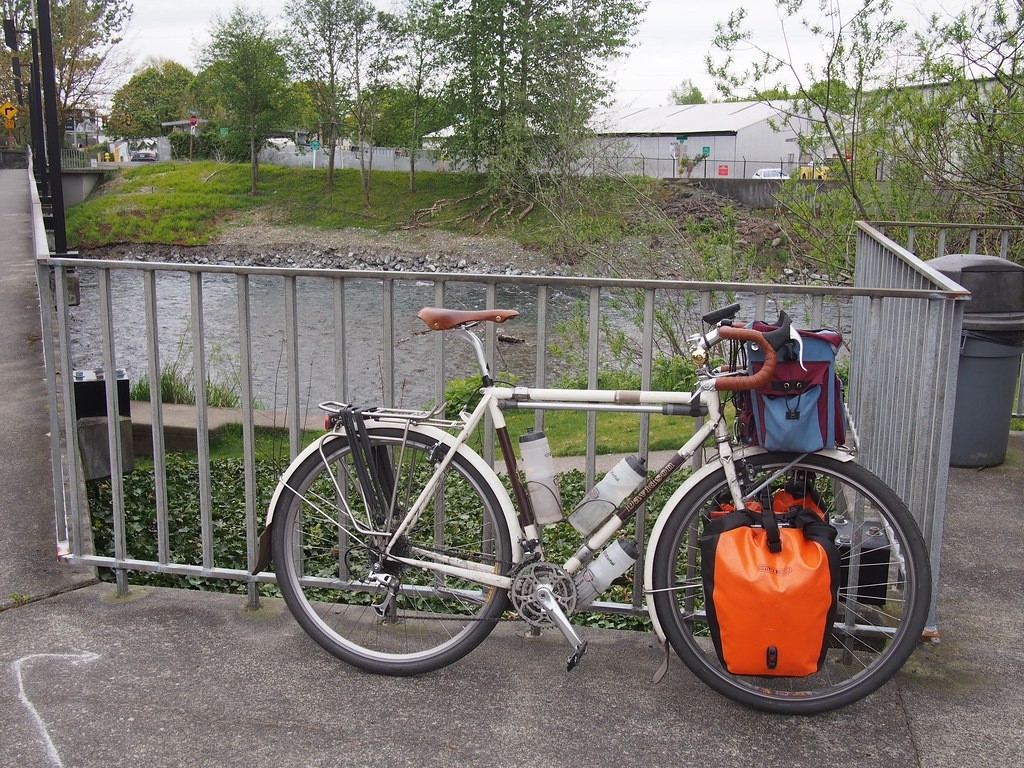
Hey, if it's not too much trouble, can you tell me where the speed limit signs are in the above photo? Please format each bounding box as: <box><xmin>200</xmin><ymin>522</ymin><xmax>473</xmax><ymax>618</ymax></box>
<box><xmin>6</xmin><ymin>120</ymin><xmax>14</xmax><ymax>129</ymax></box>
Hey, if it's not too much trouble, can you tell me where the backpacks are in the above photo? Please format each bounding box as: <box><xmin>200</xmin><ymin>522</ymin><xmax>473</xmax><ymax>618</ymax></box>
<box><xmin>740</xmin><ymin>319</ymin><xmax>847</xmax><ymax>454</ymax></box>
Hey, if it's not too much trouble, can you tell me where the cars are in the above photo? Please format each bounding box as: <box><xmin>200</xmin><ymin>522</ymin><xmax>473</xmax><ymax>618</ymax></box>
<box><xmin>131</xmin><ymin>151</ymin><xmax>158</xmax><ymax>161</ymax></box>
<box><xmin>796</xmin><ymin>157</ymin><xmax>850</xmax><ymax>180</ymax></box>
<box><xmin>752</xmin><ymin>168</ymin><xmax>791</xmax><ymax>179</ymax></box>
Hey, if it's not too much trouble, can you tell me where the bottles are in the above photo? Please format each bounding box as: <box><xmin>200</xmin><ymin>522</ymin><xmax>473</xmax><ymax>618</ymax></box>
<box><xmin>569</xmin><ymin>535</ymin><xmax>641</xmax><ymax>612</ymax></box>
<box><xmin>518</xmin><ymin>427</ymin><xmax>562</xmax><ymax>524</ymax></box>
<box><xmin>567</xmin><ymin>455</ymin><xmax>649</xmax><ymax>535</ymax></box>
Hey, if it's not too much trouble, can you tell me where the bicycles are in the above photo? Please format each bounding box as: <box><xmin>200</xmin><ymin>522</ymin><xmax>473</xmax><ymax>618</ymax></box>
<box><xmin>251</xmin><ymin>301</ymin><xmax>934</xmax><ymax>714</ymax></box>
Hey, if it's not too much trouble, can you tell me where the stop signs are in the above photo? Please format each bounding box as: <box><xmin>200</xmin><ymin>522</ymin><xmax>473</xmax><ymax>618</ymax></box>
<box><xmin>190</xmin><ymin>115</ymin><xmax>197</xmax><ymax>125</ymax></box>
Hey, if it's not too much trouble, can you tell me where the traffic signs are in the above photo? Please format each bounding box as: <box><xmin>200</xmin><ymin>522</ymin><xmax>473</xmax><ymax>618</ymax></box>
<box><xmin>191</xmin><ymin>126</ymin><xmax>195</xmax><ymax>135</ymax></box>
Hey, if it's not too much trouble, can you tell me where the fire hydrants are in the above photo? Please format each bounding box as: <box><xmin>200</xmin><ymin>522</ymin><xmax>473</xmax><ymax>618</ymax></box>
<box><xmin>104</xmin><ymin>153</ymin><xmax>110</xmax><ymax>163</ymax></box>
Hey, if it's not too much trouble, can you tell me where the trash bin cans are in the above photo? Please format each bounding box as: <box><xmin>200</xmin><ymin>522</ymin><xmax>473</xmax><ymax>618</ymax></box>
<box><xmin>923</xmin><ymin>254</ymin><xmax>1024</xmax><ymax>468</ymax></box>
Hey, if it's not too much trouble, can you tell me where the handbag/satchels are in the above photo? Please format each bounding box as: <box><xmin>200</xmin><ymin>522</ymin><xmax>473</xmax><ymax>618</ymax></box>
<box><xmin>700</xmin><ymin>473</ymin><xmax>840</xmax><ymax>678</ymax></box>
